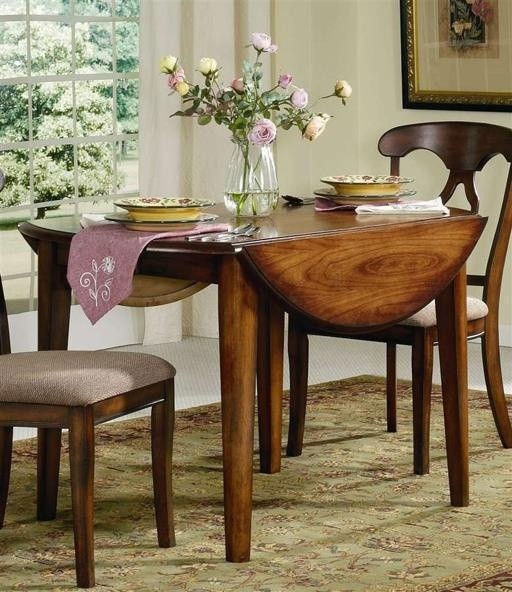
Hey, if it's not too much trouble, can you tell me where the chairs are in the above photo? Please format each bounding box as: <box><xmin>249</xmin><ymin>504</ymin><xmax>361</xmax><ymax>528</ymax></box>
<box><xmin>1</xmin><ymin>274</ymin><xmax>178</xmax><ymax>587</ymax></box>
<box><xmin>286</xmin><ymin>120</ymin><xmax>511</xmax><ymax>475</ymax></box>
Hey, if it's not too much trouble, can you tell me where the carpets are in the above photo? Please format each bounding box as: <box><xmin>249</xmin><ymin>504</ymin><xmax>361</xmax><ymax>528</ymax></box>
<box><xmin>1</xmin><ymin>374</ymin><xmax>511</xmax><ymax>591</ymax></box>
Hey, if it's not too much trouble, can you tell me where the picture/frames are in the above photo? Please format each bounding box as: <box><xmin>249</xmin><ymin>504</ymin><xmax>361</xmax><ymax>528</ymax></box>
<box><xmin>399</xmin><ymin>0</ymin><xmax>512</xmax><ymax>112</ymax></box>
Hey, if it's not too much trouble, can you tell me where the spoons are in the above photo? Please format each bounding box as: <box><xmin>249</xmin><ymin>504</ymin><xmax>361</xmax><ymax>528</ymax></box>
<box><xmin>184</xmin><ymin>222</ymin><xmax>262</xmax><ymax>241</ymax></box>
<box><xmin>281</xmin><ymin>194</ymin><xmax>315</xmax><ymax>204</ymax></box>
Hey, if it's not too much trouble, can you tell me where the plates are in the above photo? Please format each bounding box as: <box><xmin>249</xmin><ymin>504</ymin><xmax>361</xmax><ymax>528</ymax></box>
<box><xmin>320</xmin><ymin>174</ymin><xmax>415</xmax><ymax>195</ymax></box>
<box><xmin>312</xmin><ymin>187</ymin><xmax>417</xmax><ymax>206</ymax></box>
<box><xmin>114</xmin><ymin>196</ymin><xmax>217</xmax><ymax>220</ymax></box>
<box><xmin>104</xmin><ymin>211</ymin><xmax>220</xmax><ymax>232</ymax></box>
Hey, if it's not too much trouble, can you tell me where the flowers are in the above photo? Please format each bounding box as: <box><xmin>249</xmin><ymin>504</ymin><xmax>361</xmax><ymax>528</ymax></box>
<box><xmin>158</xmin><ymin>32</ymin><xmax>352</xmax><ymax>217</ymax></box>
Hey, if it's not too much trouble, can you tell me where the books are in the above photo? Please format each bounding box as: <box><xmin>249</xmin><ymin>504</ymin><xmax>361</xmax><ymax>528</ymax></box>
<box><xmin>355</xmin><ymin>196</ymin><xmax>450</xmax><ymax>219</ymax></box>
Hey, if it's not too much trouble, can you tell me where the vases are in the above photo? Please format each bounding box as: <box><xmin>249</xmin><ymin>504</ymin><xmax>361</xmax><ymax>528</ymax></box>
<box><xmin>222</xmin><ymin>135</ymin><xmax>280</xmax><ymax>217</ymax></box>
